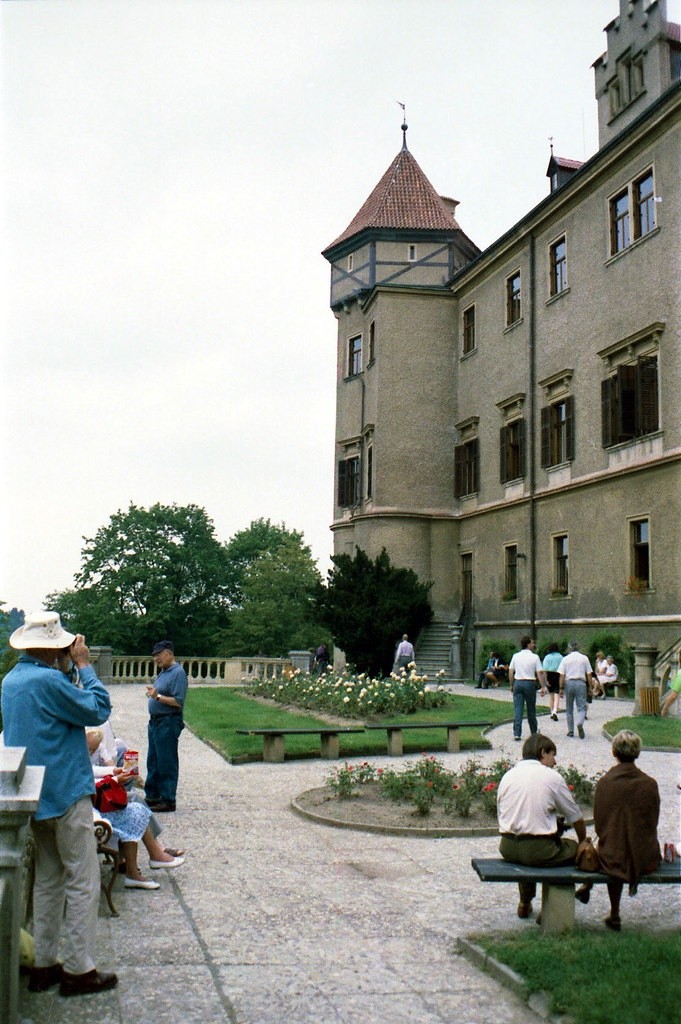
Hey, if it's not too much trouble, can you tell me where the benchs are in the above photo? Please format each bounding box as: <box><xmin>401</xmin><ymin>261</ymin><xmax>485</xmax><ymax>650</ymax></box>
<box><xmin>471</xmin><ymin>858</ymin><xmax>681</xmax><ymax>933</ymax></box>
<box><xmin>366</xmin><ymin>720</ymin><xmax>494</xmax><ymax>757</ymax></box>
<box><xmin>235</xmin><ymin>727</ymin><xmax>365</xmax><ymax>763</ymax></box>
<box><xmin>606</xmin><ymin>680</ymin><xmax>635</xmax><ymax>697</ymax></box>
<box><xmin>65</xmin><ymin>820</ymin><xmax>122</xmax><ymax>917</ymax></box>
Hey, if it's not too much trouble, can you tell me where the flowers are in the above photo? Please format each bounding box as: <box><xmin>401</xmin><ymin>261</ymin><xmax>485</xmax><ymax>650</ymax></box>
<box><xmin>626</xmin><ymin>576</ymin><xmax>648</xmax><ymax>595</ymax></box>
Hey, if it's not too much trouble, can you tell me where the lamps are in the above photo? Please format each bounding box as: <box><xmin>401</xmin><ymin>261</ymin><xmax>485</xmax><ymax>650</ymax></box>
<box><xmin>514</xmin><ymin>553</ymin><xmax>527</xmax><ymax>560</ymax></box>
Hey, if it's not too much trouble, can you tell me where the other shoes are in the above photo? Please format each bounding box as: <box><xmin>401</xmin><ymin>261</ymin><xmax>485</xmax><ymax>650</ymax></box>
<box><xmin>149</xmin><ymin>857</ymin><xmax>185</xmax><ymax>869</ymax></box>
<box><xmin>536</xmin><ymin>914</ymin><xmax>541</xmax><ymax>924</ymax></box>
<box><xmin>605</xmin><ymin>916</ymin><xmax>621</xmax><ymax>931</ymax></box>
<box><xmin>515</xmin><ymin>735</ymin><xmax>521</xmax><ymax>741</ymax></box>
<box><xmin>567</xmin><ymin>731</ymin><xmax>573</xmax><ymax>736</ymax></box>
<box><xmin>550</xmin><ymin>712</ymin><xmax>558</xmax><ymax>721</ymax></box>
<box><xmin>578</xmin><ymin>725</ymin><xmax>585</xmax><ymax>739</ymax></box>
<box><xmin>145</xmin><ymin>799</ymin><xmax>160</xmax><ymax>806</ymax></box>
<box><xmin>124</xmin><ymin>877</ymin><xmax>160</xmax><ymax>889</ymax></box>
<box><xmin>518</xmin><ymin>902</ymin><xmax>533</xmax><ymax>917</ymax></box>
<box><xmin>574</xmin><ymin>889</ymin><xmax>590</xmax><ymax>904</ymax></box>
<box><xmin>150</xmin><ymin>802</ymin><xmax>176</xmax><ymax>812</ymax></box>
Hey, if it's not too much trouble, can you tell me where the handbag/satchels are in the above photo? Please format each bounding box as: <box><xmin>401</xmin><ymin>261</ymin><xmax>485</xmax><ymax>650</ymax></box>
<box><xmin>575</xmin><ymin>836</ymin><xmax>601</xmax><ymax>871</ymax></box>
<box><xmin>93</xmin><ymin>774</ymin><xmax>128</xmax><ymax>813</ymax></box>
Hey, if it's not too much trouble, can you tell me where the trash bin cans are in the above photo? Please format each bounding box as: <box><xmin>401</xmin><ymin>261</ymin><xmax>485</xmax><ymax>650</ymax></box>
<box><xmin>639</xmin><ymin>686</ymin><xmax>659</xmax><ymax>716</ymax></box>
<box><xmin>284</xmin><ymin>665</ymin><xmax>297</xmax><ymax>677</ymax></box>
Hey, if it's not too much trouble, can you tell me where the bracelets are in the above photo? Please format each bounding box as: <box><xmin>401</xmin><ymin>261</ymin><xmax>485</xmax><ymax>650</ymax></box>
<box><xmin>156</xmin><ymin>693</ymin><xmax>161</xmax><ymax>700</ymax></box>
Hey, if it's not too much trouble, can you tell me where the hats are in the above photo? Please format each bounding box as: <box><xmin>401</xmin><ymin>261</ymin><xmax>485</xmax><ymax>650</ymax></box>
<box><xmin>151</xmin><ymin>641</ymin><xmax>174</xmax><ymax>655</ymax></box>
<box><xmin>9</xmin><ymin>612</ymin><xmax>77</xmax><ymax>649</ymax></box>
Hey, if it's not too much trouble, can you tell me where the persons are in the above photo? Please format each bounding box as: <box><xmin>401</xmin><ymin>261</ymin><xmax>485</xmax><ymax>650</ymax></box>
<box><xmin>556</xmin><ymin>638</ymin><xmax>593</xmax><ymax>739</ymax></box>
<box><xmin>0</xmin><ymin>611</ymin><xmax>119</xmax><ymax>997</ymax></box>
<box><xmin>395</xmin><ymin>634</ymin><xmax>415</xmax><ymax>673</ymax></box>
<box><xmin>85</xmin><ymin>725</ymin><xmax>185</xmax><ymax>889</ymax></box>
<box><xmin>497</xmin><ymin>732</ymin><xmax>586</xmax><ymax>924</ymax></box>
<box><xmin>591</xmin><ymin>651</ymin><xmax>618</xmax><ymax>700</ymax></box>
<box><xmin>508</xmin><ymin>636</ymin><xmax>545</xmax><ymax>741</ymax></box>
<box><xmin>661</xmin><ymin>650</ymin><xmax>681</xmax><ymax>722</ymax></box>
<box><xmin>474</xmin><ymin>646</ymin><xmax>509</xmax><ymax>689</ymax></box>
<box><xmin>316</xmin><ymin>641</ymin><xmax>330</xmax><ymax>665</ymax></box>
<box><xmin>143</xmin><ymin>639</ymin><xmax>190</xmax><ymax>812</ymax></box>
<box><xmin>575</xmin><ymin>729</ymin><xmax>663</xmax><ymax>931</ymax></box>
<box><xmin>542</xmin><ymin>644</ymin><xmax>564</xmax><ymax>721</ymax></box>
<box><xmin>309</xmin><ymin>648</ymin><xmax>314</xmax><ymax>652</ymax></box>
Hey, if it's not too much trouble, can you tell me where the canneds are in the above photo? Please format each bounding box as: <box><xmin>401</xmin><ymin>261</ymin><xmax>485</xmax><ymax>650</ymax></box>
<box><xmin>664</xmin><ymin>843</ymin><xmax>675</xmax><ymax>863</ymax></box>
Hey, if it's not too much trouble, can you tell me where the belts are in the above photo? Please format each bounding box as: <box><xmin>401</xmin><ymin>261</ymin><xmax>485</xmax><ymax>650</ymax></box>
<box><xmin>504</xmin><ymin>832</ymin><xmax>548</xmax><ymax>842</ymax></box>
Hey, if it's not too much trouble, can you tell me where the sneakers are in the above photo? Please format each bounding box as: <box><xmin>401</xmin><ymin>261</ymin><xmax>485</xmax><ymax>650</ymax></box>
<box><xmin>29</xmin><ymin>963</ymin><xmax>64</xmax><ymax>992</ymax></box>
<box><xmin>60</xmin><ymin>969</ymin><xmax>118</xmax><ymax>996</ymax></box>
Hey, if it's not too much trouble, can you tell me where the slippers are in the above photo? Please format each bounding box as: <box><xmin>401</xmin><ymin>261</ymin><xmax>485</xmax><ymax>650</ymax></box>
<box><xmin>111</xmin><ymin>862</ymin><xmax>141</xmax><ymax>875</ymax></box>
<box><xmin>164</xmin><ymin>848</ymin><xmax>186</xmax><ymax>857</ymax></box>
